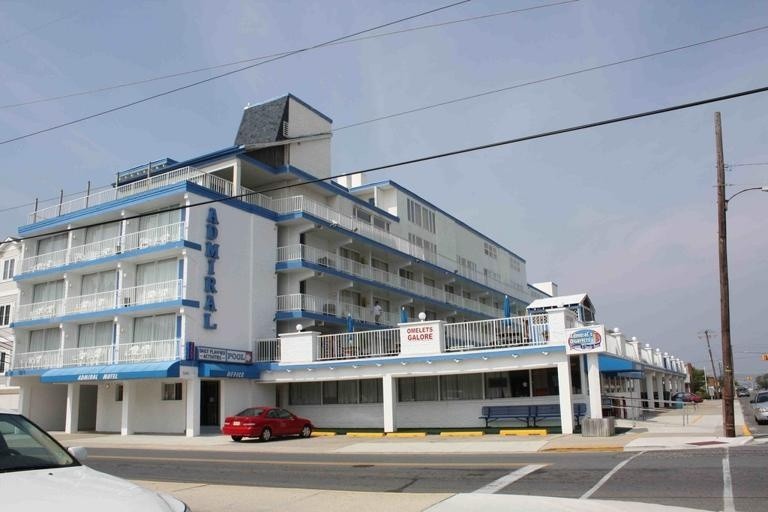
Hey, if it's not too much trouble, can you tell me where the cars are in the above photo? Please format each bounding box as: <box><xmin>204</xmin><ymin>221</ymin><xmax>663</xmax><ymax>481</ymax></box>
<box><xmin>672</xmin><ymin>392</ymin><xmax>702</xmax><ymax>403</ymax></box>
<box><xmin>0</xmin><ymin>408</ymin><xmax>191</xmax><ymax>512</ymax></box>
<box><xmin>223</xmin><ymin>407</ymin><xmax>312</xmax><ymax>442</ymax></box>
<box><xmin>737</xmin><ymin>387</ymin><xmax>768</xmax><ymax>425</ymax></box>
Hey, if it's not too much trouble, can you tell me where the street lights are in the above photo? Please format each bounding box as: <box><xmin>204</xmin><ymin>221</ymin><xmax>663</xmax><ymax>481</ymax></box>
<box><xmin>718</xmin><ymin>186</ymin><xmax>768</xmax><ymax>438</ymax></box>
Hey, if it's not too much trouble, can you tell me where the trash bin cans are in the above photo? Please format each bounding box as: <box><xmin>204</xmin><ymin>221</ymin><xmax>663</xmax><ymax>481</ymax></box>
<box><xmin>675</xmin><ymin>397</ymin><xmax>683</xmax><ymax>409</ymax></box>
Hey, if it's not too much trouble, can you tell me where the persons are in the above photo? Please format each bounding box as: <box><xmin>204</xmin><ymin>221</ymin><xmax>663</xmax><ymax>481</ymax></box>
<box><xmin>374</xmin><ymin>301</ymin><xmax>382</xmax><ymax>322</ymax></box>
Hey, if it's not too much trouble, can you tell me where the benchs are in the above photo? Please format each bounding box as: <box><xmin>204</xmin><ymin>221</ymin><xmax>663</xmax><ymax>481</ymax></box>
<box><xmin>478</xmin><ymin>403</ymin><xmax>587</xmax><ymax>431</ymax></box>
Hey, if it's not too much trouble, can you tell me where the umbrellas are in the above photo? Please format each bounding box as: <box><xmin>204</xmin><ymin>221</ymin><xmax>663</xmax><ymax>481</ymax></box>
<box><xmin>400</xmin><ymin>306</ymin><xmax>407</xmax><ymax>323</ymax></box>
<box><xmin>347</xmin><ymin>314</ymin><xmax>353</xmax><ymax>332</ymax></box>
<box><xmin>503</xmin><ymin>295</ymin><xmax>511</xmax><ymax>327</ymax></box>
